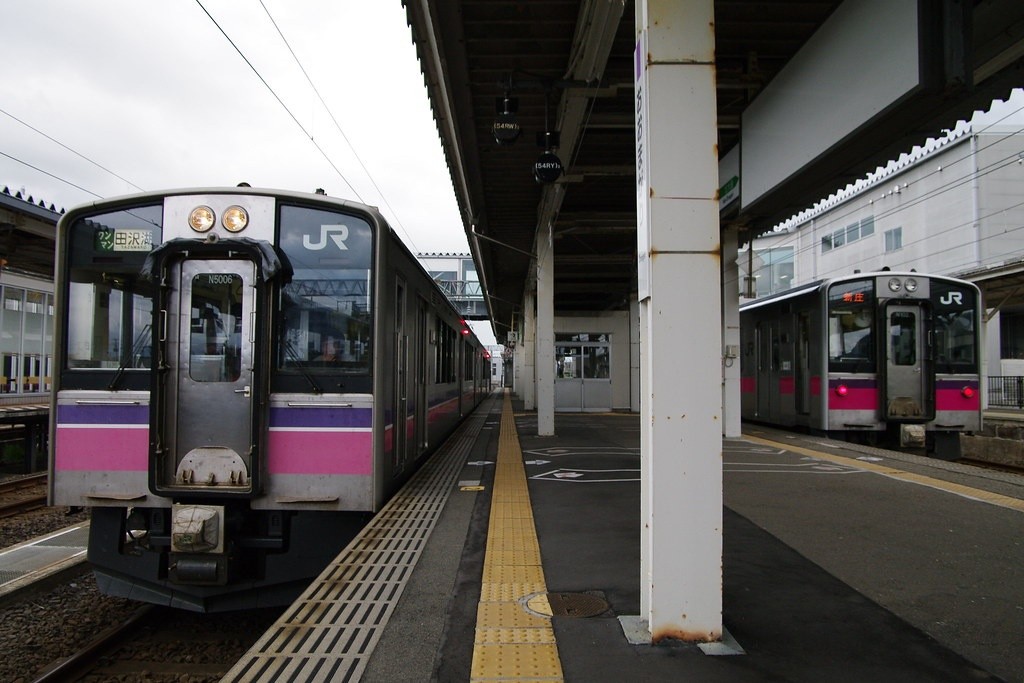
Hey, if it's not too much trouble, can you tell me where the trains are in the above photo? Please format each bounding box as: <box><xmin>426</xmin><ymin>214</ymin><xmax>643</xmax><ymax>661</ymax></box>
<box><xmin>740</xmin><ymin>267</ymin><xmax>981</xmax><ymax>460</ymax></box>
<box><xmin>47</xmin><ymin>182</ymin><xmax>491</xmax><ymax>613</ymax></box>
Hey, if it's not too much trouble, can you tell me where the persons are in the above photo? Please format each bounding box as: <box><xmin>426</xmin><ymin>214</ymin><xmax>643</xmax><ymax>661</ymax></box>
<box><xmin>311</xmin><ymin>329</ymin><xmax>357</xmax><ymax>375</ymax></box>
